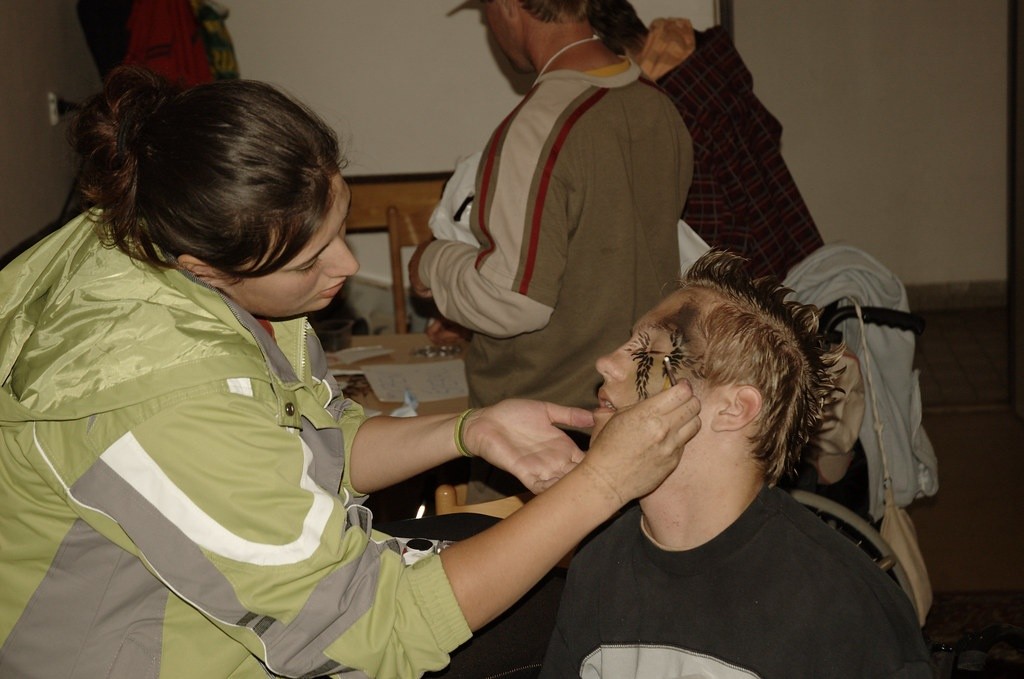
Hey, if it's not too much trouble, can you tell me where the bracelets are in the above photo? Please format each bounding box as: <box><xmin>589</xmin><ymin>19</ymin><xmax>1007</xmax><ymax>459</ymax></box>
<box><xmin>453</xmin><ymin>407</ymin><xmax>477</xmax><ymax>459</ymax></box>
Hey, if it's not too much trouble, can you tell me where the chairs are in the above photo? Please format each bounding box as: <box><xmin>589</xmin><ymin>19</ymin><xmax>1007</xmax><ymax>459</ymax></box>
<box><xmin>387</xmin><ymin>205</ymin><xmax>454</xmax><ymax>334</ymax></box>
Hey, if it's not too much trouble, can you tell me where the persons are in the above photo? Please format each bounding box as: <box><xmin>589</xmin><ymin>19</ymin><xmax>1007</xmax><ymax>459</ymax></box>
<box><xmin>585</xmin><ymin>0</ymin><xmax>830</xmax><ymax>281</ymax></box>
<box><xmin>0</xmin><ymin>64</ymin><xmax>707</xmax><ymax>679</ymax></box>
<box><xmin>540</xmin><ymin>245</ymin><xmax>941</xmax><ymax>679</ymax></box>
<box><xmin>407</xmin><ymin>0</ymin><xmax>696</xmax><ymax>505</ymax></box>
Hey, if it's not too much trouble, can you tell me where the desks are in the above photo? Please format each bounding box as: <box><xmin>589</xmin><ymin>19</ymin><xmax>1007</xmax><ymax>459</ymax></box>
<box><xmin>326</xmin><ymin>333</ymin><xmax>472</xmax><ymax>417</ymax></box>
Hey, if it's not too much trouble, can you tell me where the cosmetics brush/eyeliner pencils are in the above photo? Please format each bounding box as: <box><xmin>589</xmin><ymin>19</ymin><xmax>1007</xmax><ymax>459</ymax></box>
<box><xmin>662</xmin><ymin>353</ymin><xmax>679</xmax><ymax>388</ymax></box>
